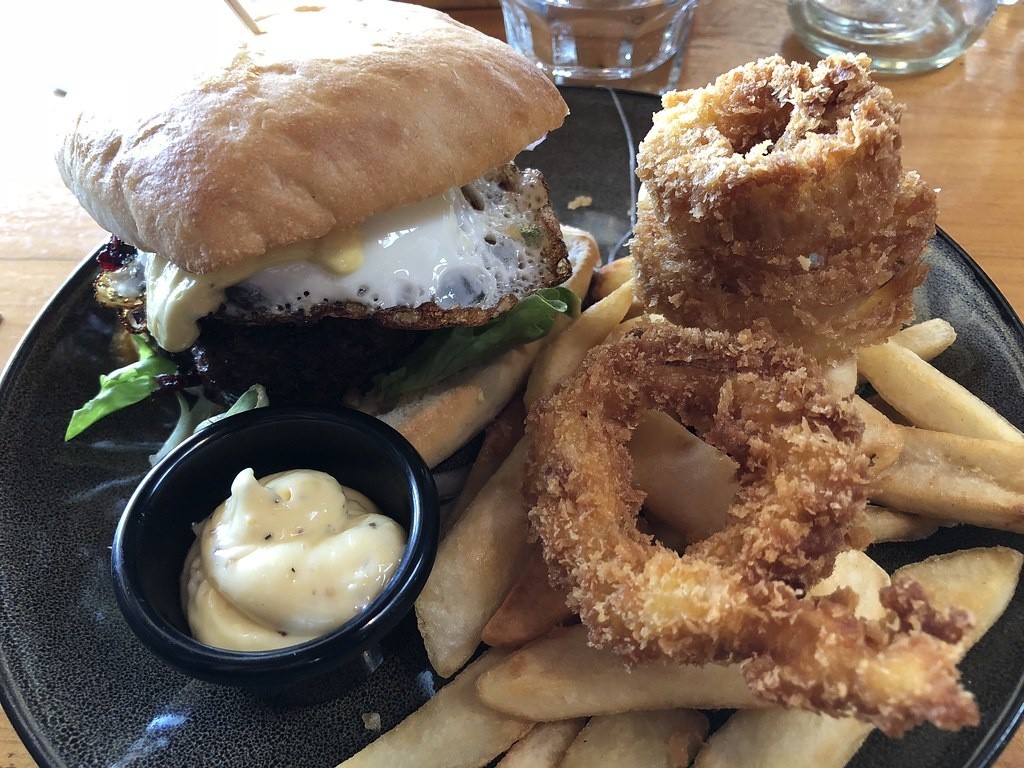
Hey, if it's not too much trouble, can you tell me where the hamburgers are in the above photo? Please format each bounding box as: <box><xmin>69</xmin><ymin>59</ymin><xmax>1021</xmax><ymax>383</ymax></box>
<box><xmin>50</xmin><ymin>1</ymin><xmax>597</xmax><ymax>424</ymax></box>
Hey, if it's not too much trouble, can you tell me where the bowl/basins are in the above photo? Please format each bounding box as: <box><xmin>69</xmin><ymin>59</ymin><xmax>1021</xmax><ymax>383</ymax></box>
<box><xmin>111</xmin><ymin>406</ymin><xmax>439</xmax><ymax>705</ymax></box>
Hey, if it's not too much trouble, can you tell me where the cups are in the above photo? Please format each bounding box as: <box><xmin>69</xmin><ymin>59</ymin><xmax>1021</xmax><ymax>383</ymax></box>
<box><xmin>787</xmin><ymin>0</ymin><xmax>1000</xmax><ymax>75</ymax></box>
<box><xmin>500</xmin><ymin>0</ymin><xmax>695</xmax><ymax>96</ymax></box>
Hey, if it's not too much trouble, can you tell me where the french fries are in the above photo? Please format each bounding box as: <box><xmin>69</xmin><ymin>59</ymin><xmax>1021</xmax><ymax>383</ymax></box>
<box><xmin>320</xmin><ymin>264</ymin><xmax>1024</xmax><ymax>768</ymax></box>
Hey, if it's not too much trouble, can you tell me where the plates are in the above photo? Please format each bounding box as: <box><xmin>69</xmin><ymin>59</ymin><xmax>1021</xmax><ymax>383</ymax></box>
<box><xmin>0</xmin><ymin>88</ymin><xmax>1024</xmax><ymax>768</ymax></box>
